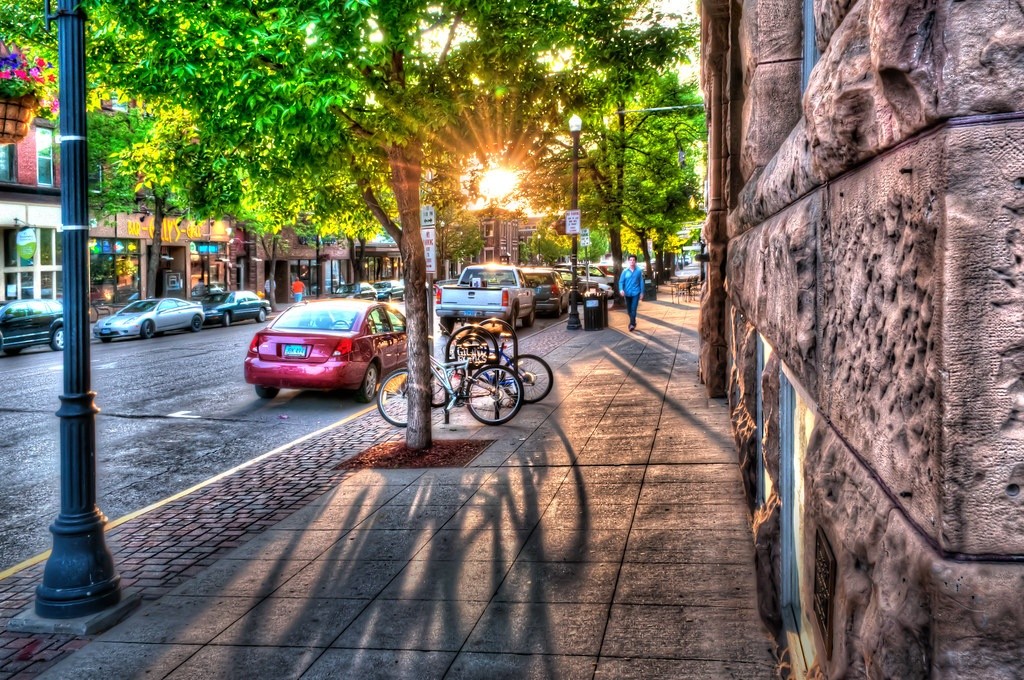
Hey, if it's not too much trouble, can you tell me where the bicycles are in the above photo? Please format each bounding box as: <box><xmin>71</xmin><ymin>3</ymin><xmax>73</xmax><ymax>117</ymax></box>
<box><xmin>429</xmin><ymin>323</ymin><xmax>553</xmax><ymax>408</ymax></box>
<box><xmin>377</xmin><ymin>335</ymin><xmax>524</xmax><ymax>426</ymax></box>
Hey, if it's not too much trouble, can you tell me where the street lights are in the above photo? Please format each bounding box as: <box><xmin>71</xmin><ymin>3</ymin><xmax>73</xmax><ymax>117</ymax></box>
<box><xmin>565</xmin><ymin>114</ymin><xmax>584</xmax><ymax>330</ymax></box>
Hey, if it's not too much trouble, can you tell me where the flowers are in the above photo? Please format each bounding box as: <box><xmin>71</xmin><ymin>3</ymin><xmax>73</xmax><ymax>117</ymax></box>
<box><xmin>0</xmin><ymin>54</ymin><xmax>60</xmax><ymax>118</ymax></box>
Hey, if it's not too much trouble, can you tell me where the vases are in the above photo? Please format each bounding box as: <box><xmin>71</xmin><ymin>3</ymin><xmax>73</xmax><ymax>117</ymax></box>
<box><xmin>0</xmin><ymin>94</ymin><xmax>39</xmax><ymax>144</ymax></box>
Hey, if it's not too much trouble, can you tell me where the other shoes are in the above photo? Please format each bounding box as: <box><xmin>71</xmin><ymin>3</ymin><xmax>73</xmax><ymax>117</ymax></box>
<box><xmin>630</xmin><ymin>323</ymin><xmax>636</xmax><ymax>331</ymax></box>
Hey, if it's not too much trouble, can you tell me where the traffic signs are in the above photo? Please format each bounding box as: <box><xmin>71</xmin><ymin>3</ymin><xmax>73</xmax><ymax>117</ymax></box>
<box><xmin>421</xmin><ymin>204</ymin><xmax>436</xmax><ymax>226</ymax></box>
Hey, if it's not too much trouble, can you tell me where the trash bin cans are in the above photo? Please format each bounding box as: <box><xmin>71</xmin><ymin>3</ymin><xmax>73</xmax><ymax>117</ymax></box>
<box><xmin>583</xmin><ymin>291</ymin><xmax>609</xmax><ymax>331</ymax></box>
<box><xmin>642</xmin><ymin>274</ymin><xmax>657</xmax><ymax>301</ymax></box>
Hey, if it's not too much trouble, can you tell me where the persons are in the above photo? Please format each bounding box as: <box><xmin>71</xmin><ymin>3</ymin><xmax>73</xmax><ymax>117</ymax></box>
<box><xmin>292</xmin><ymin>277</ymin><xmax>306</xmax><ymax>303</ymax></box>
<box><xmin>191</xmin><ymin>278</ymin><xmax>207</xmax><ymax>297</ymax></box>
<box><xmin>618</xmin><ymin>254</ymin><xmax>645</xmax><ymax>332</ymax></box>
<box><xmin>265</xmin><ymin>280</ymin><xmax>276</xmax><ymax>301</ymax></box>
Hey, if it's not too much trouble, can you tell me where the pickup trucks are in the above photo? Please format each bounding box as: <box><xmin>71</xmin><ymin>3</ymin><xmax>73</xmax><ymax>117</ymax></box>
<box><xmin>436</xmin><ymin>265</ymin><xmax>536</xmax><ymax>336</ymax></box>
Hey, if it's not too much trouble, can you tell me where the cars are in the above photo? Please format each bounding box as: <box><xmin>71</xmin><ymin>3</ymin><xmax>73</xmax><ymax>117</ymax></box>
<box><xmin>372</xmin><ymin>279</ymin><xmax>406</xmax><ymax>303</ymax></box>
<box><xmin>330</xmin><ymin>282</ymin><xmax>378</xmax><ymax>302</ymax></box>
<box><xmin>434</xmin><ymin>279</ymin><xmax>459</xmax><ymax>287</ymax></box>
<box><xmin>552</xmin><ymin>268</ymin><xmax>598</xmax><ymax>304</ymax></box>
<box><xmin>244</xmin><ymin>300</ymin><xmax>408</xmax><ymax>403</ymax></box>
<box><xmin>93</xmin><ymin>298</ymin><xmax>205</xmax><ymax>343</ymax></box>
<box><xmin>199</xmin><ymin>291</ymin><xmax>272</xmax><ymax>326</ymax></box>
<box><xmin>0</xmin><ymin>298</ymin><xmax>65</xmax><ymax>355</ymax></box>
<box><xmin>520</xmin><ymin>268</ymin><xmax>570</xmax><ymax>318</ymax></box>
<box><xmin>598</xmin><ymin>266</ymin><xmax>624</xmax><ymax>279</ymax></box>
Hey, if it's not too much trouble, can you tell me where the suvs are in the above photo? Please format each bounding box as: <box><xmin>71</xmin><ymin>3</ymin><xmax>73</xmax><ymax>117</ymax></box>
<box><xmin>554</xmin><ymin>264</ymin><xmax>615</xmax><ymax>291</ymax></box>
